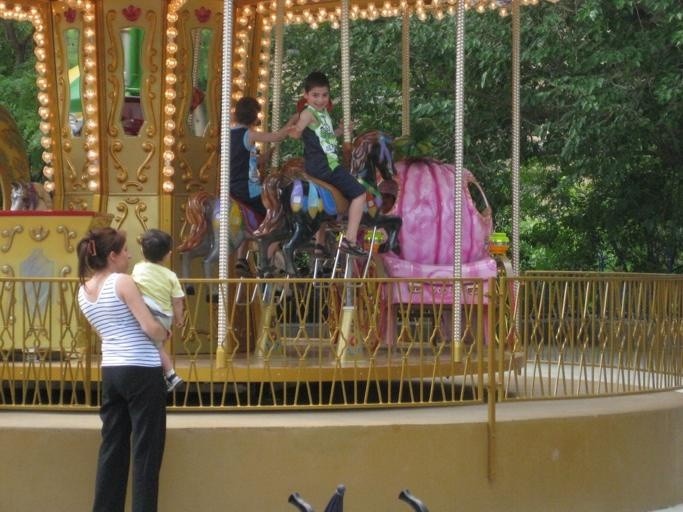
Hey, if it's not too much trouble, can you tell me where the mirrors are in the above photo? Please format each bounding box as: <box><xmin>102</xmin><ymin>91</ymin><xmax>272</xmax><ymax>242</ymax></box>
<box><xmin>64</xmin><ymin>27</ymin><xmax>220</xmax><ymax>137</ymax></box>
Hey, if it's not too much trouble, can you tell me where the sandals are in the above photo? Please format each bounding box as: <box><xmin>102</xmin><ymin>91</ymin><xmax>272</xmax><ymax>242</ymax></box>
<box><xmin>313</xmin><ymin>242</ymin><xmax>333</xmax><ymax>259</ymax></box>
<box><xmin>233</xmin><ymin>257</ymin><xmax>251</xmax><ymax>276</ymax></box>
<box><xmin>339</xmin><ymin>236</ymin><xmax>369</xmax><ymax>257</ymax></box>
<box><xmin>166</xmin><ymin>374</ymin><xmax>184</xmax><ymax>393</ymax></box>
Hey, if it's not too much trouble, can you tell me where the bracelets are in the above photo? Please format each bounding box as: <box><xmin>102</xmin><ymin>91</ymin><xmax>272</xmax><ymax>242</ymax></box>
<box><xmin>165</xmin><ymin>328</ymin><xmax>171</xmax><ymax>341</ymax></box>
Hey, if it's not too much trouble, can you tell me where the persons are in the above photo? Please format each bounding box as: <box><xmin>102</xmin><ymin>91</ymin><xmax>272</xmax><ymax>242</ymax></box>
<box><xmin>76</xmin><ymin>226</ymin><xmax>171</xmax><ymax>512</ymax></box>
<box><xmin>229</xmin><ymin>95</ymin><xmax>298</xmax><ymax>276</ymax></box>
<box><xmin>130</xmin><ymin>230</ymin><xmax>187</xmax><ymax>393</ymax></box>
<box><xmin>286</xmin><ymin>71</ymin><xmax>368</xmax><ymax>258</ymax></box>
